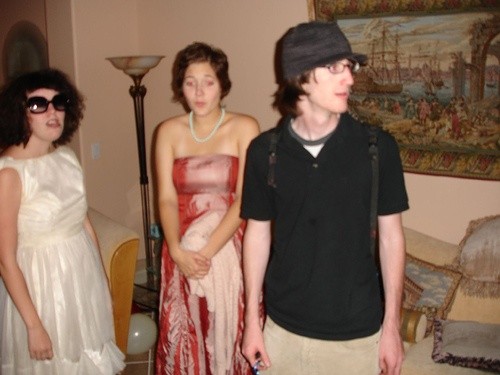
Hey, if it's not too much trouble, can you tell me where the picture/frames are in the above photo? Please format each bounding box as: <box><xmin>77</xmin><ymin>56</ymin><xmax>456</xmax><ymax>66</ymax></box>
<box><xmin>309</xmin><ymin>0</ymin><xmax>500</xmax><ymax>181</ymax></box>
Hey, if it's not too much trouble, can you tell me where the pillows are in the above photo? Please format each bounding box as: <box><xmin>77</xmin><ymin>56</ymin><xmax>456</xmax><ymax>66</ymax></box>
<box><xmin>454</xmin><ymin>214</ymin><xmax>500</xmax><ymax>298</ymax></box>
<box><xmin>432</xmin><ymin>320</ymin><xmax>500</xmax><ymax>370</ymax></box>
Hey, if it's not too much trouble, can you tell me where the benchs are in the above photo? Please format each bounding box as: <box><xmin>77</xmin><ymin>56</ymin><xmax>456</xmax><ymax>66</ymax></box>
<box><xmin>86</xmin><ymin>207</ymin><xmax>139</xmax><ymax>355</ymax></box>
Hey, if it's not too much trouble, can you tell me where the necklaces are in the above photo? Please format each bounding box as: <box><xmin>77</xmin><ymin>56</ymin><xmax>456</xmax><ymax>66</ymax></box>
<box><xmin>188</xmin><ymin>107</ymin><xmax>225</xmax><ymax>142</ymax></box>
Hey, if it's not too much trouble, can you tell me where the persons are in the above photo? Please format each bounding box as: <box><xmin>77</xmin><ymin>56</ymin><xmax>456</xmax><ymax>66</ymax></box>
<box><xmin>0</xmin><ymin>69</ymin><xmax>126</xmax><ymax>375</ymax></box>
<box><xmin>239</xmin><ymin>19</ymin><xmax>409</xmax><ymax>375</ymax></box>
<box><xmin>154</xmin><ymin>41</ymin><xmax>266</xmax><ymax>375</ymax></box>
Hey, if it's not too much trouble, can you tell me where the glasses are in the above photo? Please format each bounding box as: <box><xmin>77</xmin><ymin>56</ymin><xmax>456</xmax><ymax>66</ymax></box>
<box><xmin>315</xmin><ymin>62</ymin><xmax>360</xmax><ymax>74</ymax></box>
<box><xmin>27</xmin><ymin>94</ymin><xmax>71</xmax><ymax>114</ymax></box>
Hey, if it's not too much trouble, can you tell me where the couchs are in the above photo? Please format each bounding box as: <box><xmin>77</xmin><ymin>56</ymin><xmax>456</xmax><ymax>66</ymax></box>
<box><xmin>402</xmin><ymin>226</ymin><xmax>500</xmax><ymax>375</ymax></box>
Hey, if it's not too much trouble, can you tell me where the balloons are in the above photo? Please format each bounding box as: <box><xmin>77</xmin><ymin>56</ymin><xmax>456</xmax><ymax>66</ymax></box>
<box><xmin>127</xmin><ymin>313</ymin><xmax>157</xmax><ymax>354</ymax></box>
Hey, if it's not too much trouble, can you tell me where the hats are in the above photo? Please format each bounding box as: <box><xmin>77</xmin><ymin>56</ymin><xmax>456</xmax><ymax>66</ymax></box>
<box><xmin>274</xmin><ymin>22</ymin><xmax>368</xmax><ymax>84</ymax></box>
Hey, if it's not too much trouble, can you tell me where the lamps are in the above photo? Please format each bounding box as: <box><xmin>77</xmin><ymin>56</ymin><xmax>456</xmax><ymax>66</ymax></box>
<box><xmin>105</xmin><ymin>54</ymin><xmax>166</xmax><ymax>289</ymax></box>
